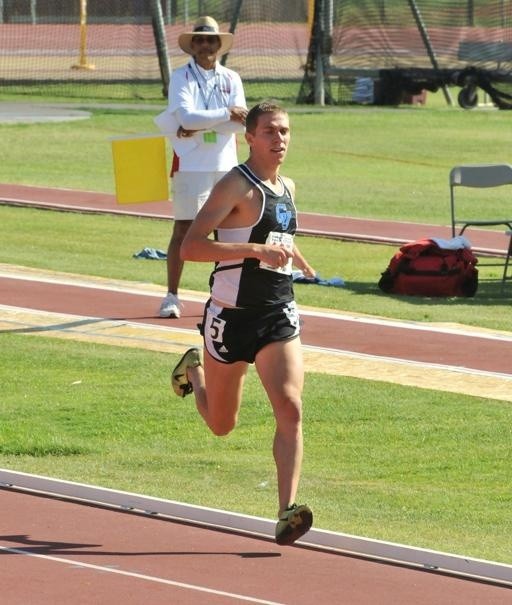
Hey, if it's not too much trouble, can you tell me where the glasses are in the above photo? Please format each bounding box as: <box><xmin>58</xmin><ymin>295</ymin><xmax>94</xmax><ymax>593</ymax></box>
<box><xmin>192</xmin><ymin>36</ymin><xmax>216</xmax><ymax>44</ymax></box>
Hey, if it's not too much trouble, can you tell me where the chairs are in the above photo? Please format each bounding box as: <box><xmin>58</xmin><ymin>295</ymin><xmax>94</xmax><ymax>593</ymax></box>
<box><xmin>449</xmin><ymin>164</ymin><xmax>512</xmax><ymax>295</ymax></box>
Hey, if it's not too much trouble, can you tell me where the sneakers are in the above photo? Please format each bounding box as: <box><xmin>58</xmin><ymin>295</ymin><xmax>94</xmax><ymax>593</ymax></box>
<box><xmin>172</xmin><ymin>349</ymin><xmax>204</xmax><ymax>397</ymax></box>
<box><xmin>160</xmin><ymin>292</ymin><xmax>180</xmax><ymax>318</ymax></box>
<box><xmin>275</xmin><ymin>503</ymin><xmax>312</xmax><ymax>545</ymax></box>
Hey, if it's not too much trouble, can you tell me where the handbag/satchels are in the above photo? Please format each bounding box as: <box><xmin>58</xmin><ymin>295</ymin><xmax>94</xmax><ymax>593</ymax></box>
<box><xmin>378</xmin><ymin>240</ymin><xmax>478</xmax><ymax>296</ymax></box>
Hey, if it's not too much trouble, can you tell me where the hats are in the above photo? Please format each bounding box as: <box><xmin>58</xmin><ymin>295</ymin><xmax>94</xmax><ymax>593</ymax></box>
<box><xmin>179</xmin><ymin>16</ymin><xmax>233</xmax><ymax>56</ymax></box>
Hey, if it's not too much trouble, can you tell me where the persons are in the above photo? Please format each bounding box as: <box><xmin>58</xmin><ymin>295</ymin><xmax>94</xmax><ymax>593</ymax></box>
<box><xmin>171</xmin><ymin>102</ymin><xmax>317</xmax><ymax>546</ymax></box>
<box><xmin>153</xmin><ymin>15</ymin><xmax>250</xmax><ymax>319</ymax></box>
